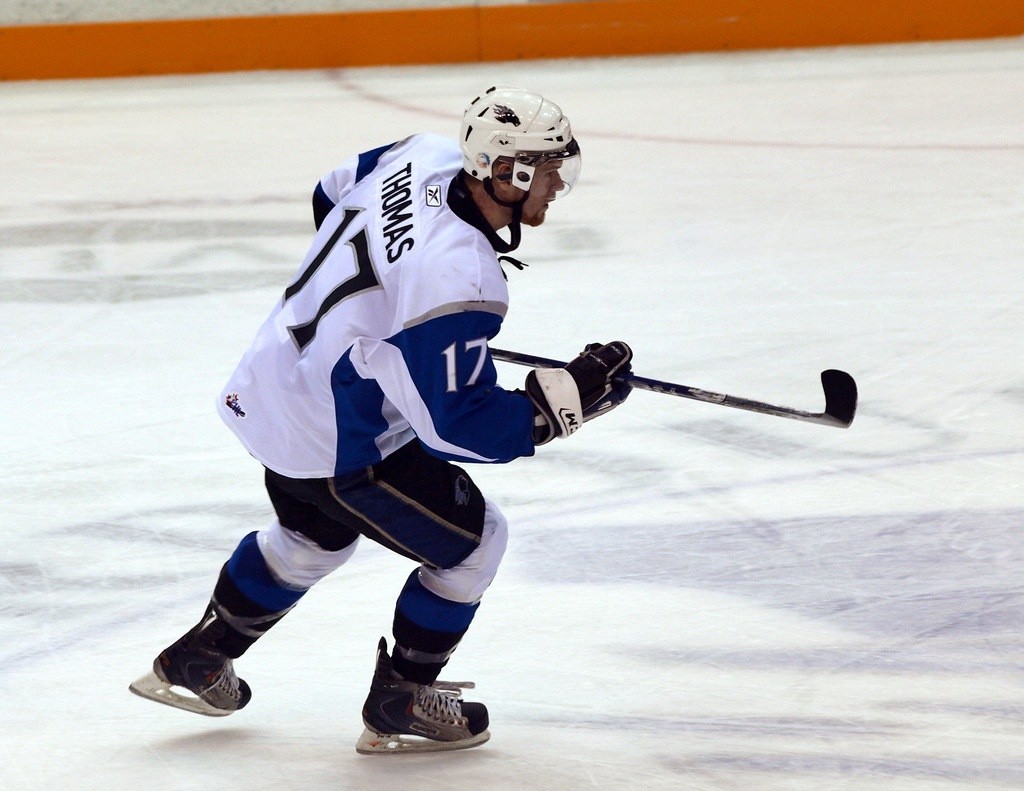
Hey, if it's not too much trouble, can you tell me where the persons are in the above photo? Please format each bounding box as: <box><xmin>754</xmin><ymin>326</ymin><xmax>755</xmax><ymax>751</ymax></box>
<box><xmin>128</xmin><ymin>85</ymin><xmax>634</xmax><ymax>755</ymax></box>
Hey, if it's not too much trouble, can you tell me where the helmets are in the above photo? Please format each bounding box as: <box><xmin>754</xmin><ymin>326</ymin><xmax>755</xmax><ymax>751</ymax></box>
<box><xmin>459</xmin><ymin>86</ymin><xmax>585</xmax><ymax>201</ymax></box>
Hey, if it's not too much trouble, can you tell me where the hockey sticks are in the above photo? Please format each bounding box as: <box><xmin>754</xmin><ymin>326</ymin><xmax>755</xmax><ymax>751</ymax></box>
<box><xmin>489</xmin><ymin>347</ymin><xmax>857</xmax><ymax>426</ymax></box>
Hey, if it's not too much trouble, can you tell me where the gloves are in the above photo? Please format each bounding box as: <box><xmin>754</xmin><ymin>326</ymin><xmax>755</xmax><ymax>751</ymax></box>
<box><xmin>520</xmin><ymin>341</ymin><xmax>636</xmax><ymax>445</ymax></box>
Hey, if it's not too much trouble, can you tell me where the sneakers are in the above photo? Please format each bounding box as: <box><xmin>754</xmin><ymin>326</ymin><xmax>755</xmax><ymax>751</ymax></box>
<box><xmin>355</xmin><ymin>636</ymin><xmax>492</xmax><ymax>753</ymax></box>
<box><xmin>128</xmin><ymin>596</ymin><xmax>253</xmax><ymax>716</ymax></box>
